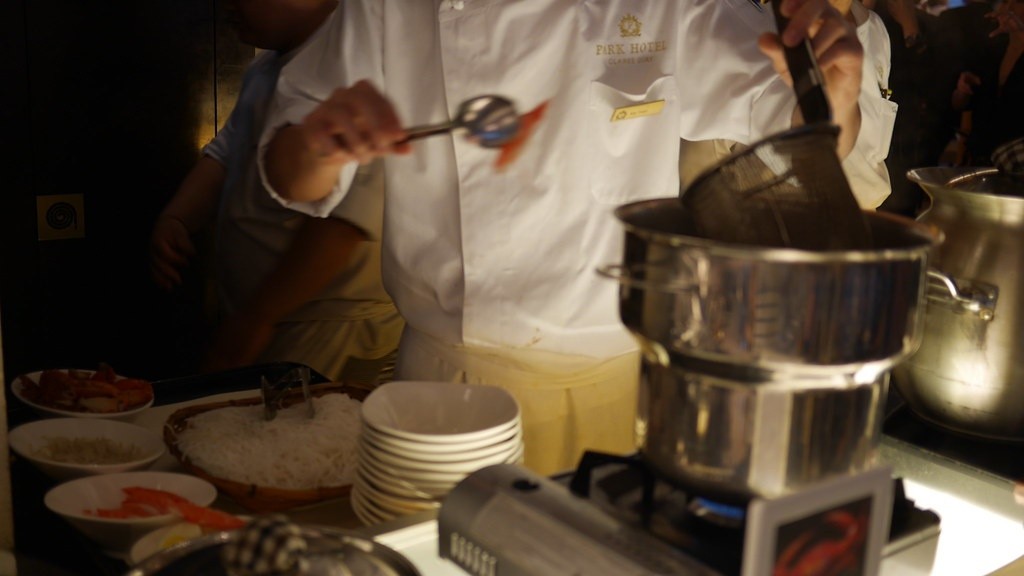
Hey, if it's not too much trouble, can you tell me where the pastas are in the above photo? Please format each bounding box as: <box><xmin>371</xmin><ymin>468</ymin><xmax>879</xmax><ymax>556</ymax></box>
<box><xmin>175</xmin><ymin>394</ymin><xmax>366</xmax><ymax>489</ymax></box>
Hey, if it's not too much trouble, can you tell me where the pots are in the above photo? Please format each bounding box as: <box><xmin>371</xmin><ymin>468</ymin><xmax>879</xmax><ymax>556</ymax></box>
<box><xmin>887</xmin><ymin>166</ymin><xmax>1024</xmax><ymax>442</ymax></box>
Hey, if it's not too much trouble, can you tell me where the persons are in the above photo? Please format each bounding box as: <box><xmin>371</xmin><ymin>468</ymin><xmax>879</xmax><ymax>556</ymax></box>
<box><xmin>147</xmin><ymin>1</ymin><xmax>412</xmax><ymax>408</ymax></box>
<box><xmin>861</xmin><ymin>0</ymin><xmax>1024</xmax><ymax>238</ymax></box>
<box><xmin>254</xmin><ymin>1</ymin><xmax>900</xmax><ymax>490</ymax></box>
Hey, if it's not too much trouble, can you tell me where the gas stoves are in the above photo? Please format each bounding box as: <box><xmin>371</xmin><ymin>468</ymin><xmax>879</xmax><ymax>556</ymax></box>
<box><xmin>437</xmin><ymin>446</ymin><xmax>942</xmax><ymax>576</ymax></box>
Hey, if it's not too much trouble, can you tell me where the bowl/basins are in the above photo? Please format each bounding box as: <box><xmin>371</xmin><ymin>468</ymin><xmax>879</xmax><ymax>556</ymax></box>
<box><xmin>129</xmin><ymin>516</ymin><xmax>262</xmax><ymax>568</ymax></box>
<box><xmin>11</xmin><ymin>369</ymin><xmax>155</xmax><ymax>418</ymax></box>
<box><xmin>8</xmin><ymin>419</ymin><xmax>168</xmax><ymax>479</ymax></box>
<box><xmin>349</xmin><ymin>382</ymin><xmax>526</xmax><ymax>527</ymax></box>
<box><xmin>43</xmin><ymin>473</ymin><xmax>217</xmax><ymax>559</ymax></box>
<box><xmin>131</xmin><ymin>530</ymin><xmax>420</xmax><ymax>576</ymax></box>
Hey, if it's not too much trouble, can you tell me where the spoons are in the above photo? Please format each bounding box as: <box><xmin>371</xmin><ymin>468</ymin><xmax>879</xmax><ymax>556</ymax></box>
<box><xmin>393</xmin><ymin>95</ymin><xmax>524</xmax><ymax>149</ymax></box>
